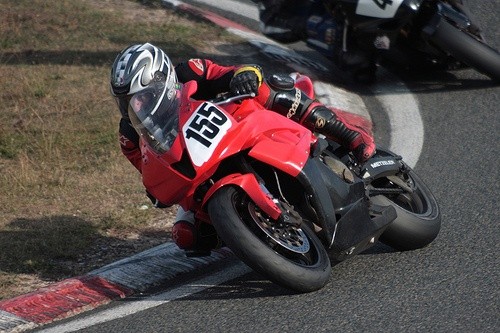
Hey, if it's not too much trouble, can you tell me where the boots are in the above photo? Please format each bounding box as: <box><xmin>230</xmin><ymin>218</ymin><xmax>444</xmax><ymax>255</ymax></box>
<box><xmin>304</xmin><ymin>104</ymin><xmax>377</xmax><ymax>162</ymax></box>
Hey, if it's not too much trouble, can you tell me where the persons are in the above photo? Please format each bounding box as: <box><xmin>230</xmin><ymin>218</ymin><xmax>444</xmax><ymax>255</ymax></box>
<box><xmin>108</xmin><ymin>42</ymin><xmax>376</xmax><ymax>250</ymax></box>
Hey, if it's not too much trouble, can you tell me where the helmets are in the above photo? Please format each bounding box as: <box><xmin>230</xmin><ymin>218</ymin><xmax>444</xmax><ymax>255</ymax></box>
<box><xmin>110</xmin><ymin>42</ymin><xmax>178</xmax><ymax>130</ymax></box>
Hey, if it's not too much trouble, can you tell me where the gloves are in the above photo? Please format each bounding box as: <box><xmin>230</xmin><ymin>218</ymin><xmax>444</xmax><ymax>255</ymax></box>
<box><xmin>307</xmin><ymin>16</ymin><xmax>344</xmax><ymax>43</ymax></box>
<box><xmin>229</xmin><ymin>70</ymin><xmax>259</xmax><ymax>103</ymax></box>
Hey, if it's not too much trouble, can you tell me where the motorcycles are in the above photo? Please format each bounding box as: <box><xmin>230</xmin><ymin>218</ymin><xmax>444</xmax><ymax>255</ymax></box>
<box><xmin>135</xmin><ymin>71</ymin><xmax>443</xmax><ymax>292</ymax></box>
<box><xmin>261</xmin><ymin>0</ymin><xmax>500</xmax><ymax>87</ymax></box>
